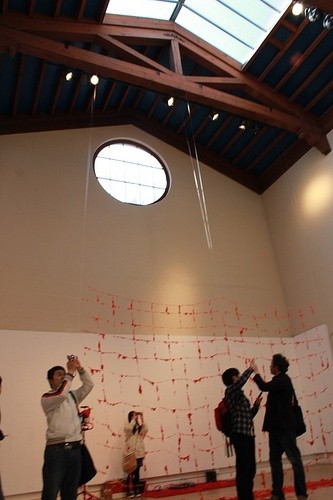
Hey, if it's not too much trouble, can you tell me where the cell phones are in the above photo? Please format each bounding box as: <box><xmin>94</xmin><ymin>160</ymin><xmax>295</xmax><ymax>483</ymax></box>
<box><xmin>135</xmin><ymin>412</ymin><xmax>141</xmax><ymax>415</ymax></box>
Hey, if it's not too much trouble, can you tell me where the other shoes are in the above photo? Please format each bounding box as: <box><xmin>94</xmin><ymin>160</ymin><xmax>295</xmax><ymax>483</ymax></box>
<box><xmin>126</xmin><ymin>492</ymin><xmax>142</xmax><ymax>499</ymax></box>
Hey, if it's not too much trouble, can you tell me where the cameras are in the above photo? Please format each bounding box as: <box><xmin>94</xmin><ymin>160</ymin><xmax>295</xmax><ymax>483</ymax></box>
<box><xmin>67</xmin><ymin>354</ymin><xmax>75</xmax><ymax>360</ymax></box>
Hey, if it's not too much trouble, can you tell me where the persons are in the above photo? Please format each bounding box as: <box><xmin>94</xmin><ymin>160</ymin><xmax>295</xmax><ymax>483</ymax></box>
<box><xmin>123</xmin><ymin>410</ymin><xmax>150</xmax><ymax>499</ymax></box>
<box><xmin>41</xmin><ymin>354</ymin><xmax>93</xmax><ymax>500</ymax></box>
<box><xmin>222</xmin><ymin>358</ymin><xmax>263</xmax><ymax>500</ymax></box>
<box><xmin>245</xmin><ymin>354</ymin><xmax>308</xmax><ymax>500</ymax></box>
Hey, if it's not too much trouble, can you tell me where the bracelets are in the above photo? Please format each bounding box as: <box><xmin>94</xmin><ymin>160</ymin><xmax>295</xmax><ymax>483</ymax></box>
<box><xmin>66</xmin><ymin>372</ymin><xmax>75</xmax><ymax>378</ymax></box>
<box><xmin>78</xmin><ymin>367</ymin><xmax>84</xmax><ymax>373</ymax></box>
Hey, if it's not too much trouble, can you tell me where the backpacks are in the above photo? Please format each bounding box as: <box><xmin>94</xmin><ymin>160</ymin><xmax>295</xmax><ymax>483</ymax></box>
<box><xmin>214</xmin><ymin>399</ymin><xmax>233</xmax><ymax>458</ymax></box>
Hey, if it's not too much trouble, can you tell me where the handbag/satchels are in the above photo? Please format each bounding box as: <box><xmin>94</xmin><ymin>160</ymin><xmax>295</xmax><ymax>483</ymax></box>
<box><xmin>121</xmin><ymin>452</ymin><xmax>137</xmax><ymax>474</ymax></box>
<box><xmin>53</xmin><ymin>443</ymin><xmax>97</xmax><ymax>495</ymax></box>
<box><xmin>291</xmin><ymin>405</ymin><xmax>306</xmax><ymax>439</ymax></box>
